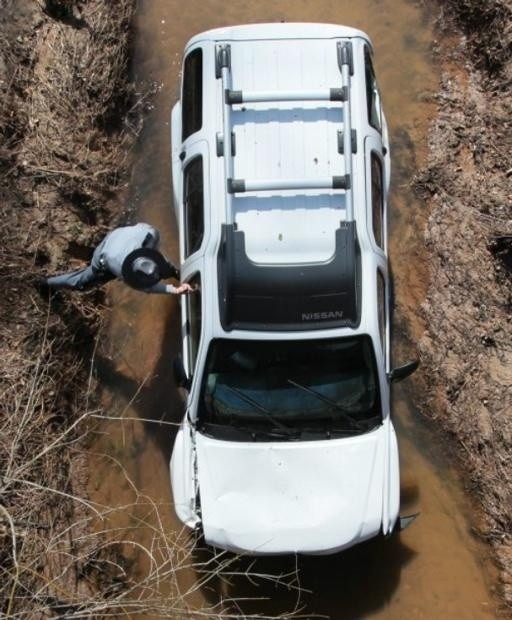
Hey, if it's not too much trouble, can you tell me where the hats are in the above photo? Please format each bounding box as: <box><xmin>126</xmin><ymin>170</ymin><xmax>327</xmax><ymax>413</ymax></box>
<box><xmin>122</xmin><ymin>248</ymin><xmax>169</xmax><ymax>289</ymax></box>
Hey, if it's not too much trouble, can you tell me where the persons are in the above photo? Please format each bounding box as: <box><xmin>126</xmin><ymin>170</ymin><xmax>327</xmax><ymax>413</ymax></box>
<box><xmin>39</xmin><ymin>223</ymin><xmax>195</xmax><ymax>300</ymax></box>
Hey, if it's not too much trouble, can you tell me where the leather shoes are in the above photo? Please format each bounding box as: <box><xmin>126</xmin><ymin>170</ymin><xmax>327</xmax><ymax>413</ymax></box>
<box><xmin>33</xmin><ymin>276</ymin><xmax>50</xmax><ymax>303</ymax></box>
<box><xmin>171</xmin><ymin>268</ymin><xmax>180</xmax><ymax>280</ymax></box>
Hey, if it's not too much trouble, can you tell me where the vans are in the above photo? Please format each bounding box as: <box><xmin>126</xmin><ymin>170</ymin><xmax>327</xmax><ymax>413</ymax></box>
<box><xmin>160</xmin><ymin>17</ymin><xmax>425</xmax><ymax>559</ymax></box>
